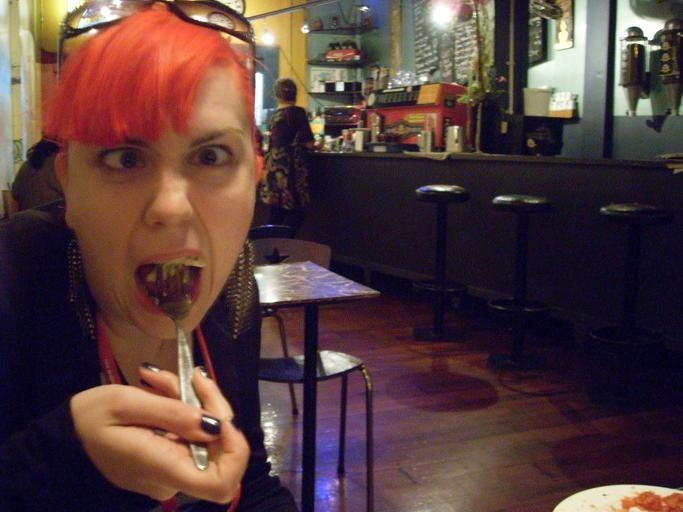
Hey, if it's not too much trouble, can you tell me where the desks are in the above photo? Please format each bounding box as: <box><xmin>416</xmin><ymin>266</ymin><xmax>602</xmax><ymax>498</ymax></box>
<box><xmin>253</xmin><ymin>258</ymin><xmax>381</xmax><ymax>465</ymax></box>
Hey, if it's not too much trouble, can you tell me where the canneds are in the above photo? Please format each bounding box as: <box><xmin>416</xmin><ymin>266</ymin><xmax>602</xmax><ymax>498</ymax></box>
<box><xmin>341</xmin><ymin>129</ymin><xmax>352</xmax><ymax>149</ymax></box>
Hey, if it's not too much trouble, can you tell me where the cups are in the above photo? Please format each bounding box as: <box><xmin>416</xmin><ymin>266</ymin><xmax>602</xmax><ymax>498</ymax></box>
<box><xmin>445</xmin><ymin>124</ymin><xmax>462</xmax><ymax>154</ymax></box>
<box><xmin>417</xmin><ymin>131</ymin><xmax>433</xmax><ymax>154</ymax></box>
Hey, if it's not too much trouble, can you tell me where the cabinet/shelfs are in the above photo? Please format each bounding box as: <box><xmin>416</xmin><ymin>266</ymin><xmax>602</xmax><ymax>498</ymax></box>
<box><xmin>307</xmin><ymin>23</ymin><xmax>380</xmax><ymax>128</ymax></box>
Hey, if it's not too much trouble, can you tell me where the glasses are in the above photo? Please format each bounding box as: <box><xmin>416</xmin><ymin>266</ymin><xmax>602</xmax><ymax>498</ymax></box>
<box><xmin>56</xmin><ymin>0</ymin><xmax>256</xmax><ymax>92</ymax></box>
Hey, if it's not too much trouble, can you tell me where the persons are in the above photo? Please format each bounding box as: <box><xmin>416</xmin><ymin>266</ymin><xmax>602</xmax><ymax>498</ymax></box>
<box><xmin>258</xmin><ymin>77</ymin><xmax>327</xmax><ymax>229</ymax></box>
<box><xmin>1</xmin><ymin>2</ymin><xmax>298</xmax><ymax>508</ymax></box>
<box><xmin>10</xmin><ymin>138</ymin><xmax>64</xmax><ymax>211</ymax></box>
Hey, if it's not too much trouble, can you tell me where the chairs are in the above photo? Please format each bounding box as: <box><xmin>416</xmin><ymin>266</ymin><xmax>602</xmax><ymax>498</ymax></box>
<box><xmin>248</xmin><ymin>223</ymin><xmax>373</xmax><ymax>511</ymax></box>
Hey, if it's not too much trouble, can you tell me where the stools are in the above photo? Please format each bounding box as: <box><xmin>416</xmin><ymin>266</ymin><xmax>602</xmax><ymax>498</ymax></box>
<box><xmin>590</xmin><ymin>201</ymin><xmax>663</xmax><ymax>352</ymax></box>
<box><xmin>485</xmin><ymin>191</ymin><xmax>555</xmax><ymax>372</ymax></box>
<box><xmin>416</xmin><ymin>186</ymin><xmax>472</xmax><ymax>343</ymax></box>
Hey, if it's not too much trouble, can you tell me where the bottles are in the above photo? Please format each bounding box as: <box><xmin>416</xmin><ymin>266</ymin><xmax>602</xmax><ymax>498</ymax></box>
<box><xmin>341</xmin><ymin>128</ymin><xmax>354</xmax><ymax>152</ymax></box>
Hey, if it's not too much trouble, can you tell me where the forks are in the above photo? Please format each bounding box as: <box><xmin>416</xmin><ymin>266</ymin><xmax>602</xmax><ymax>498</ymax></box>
<box><xmin>155</xmin><ymin>264</ymin><xmax>209</xmax><ymax>470</ymax></box>
